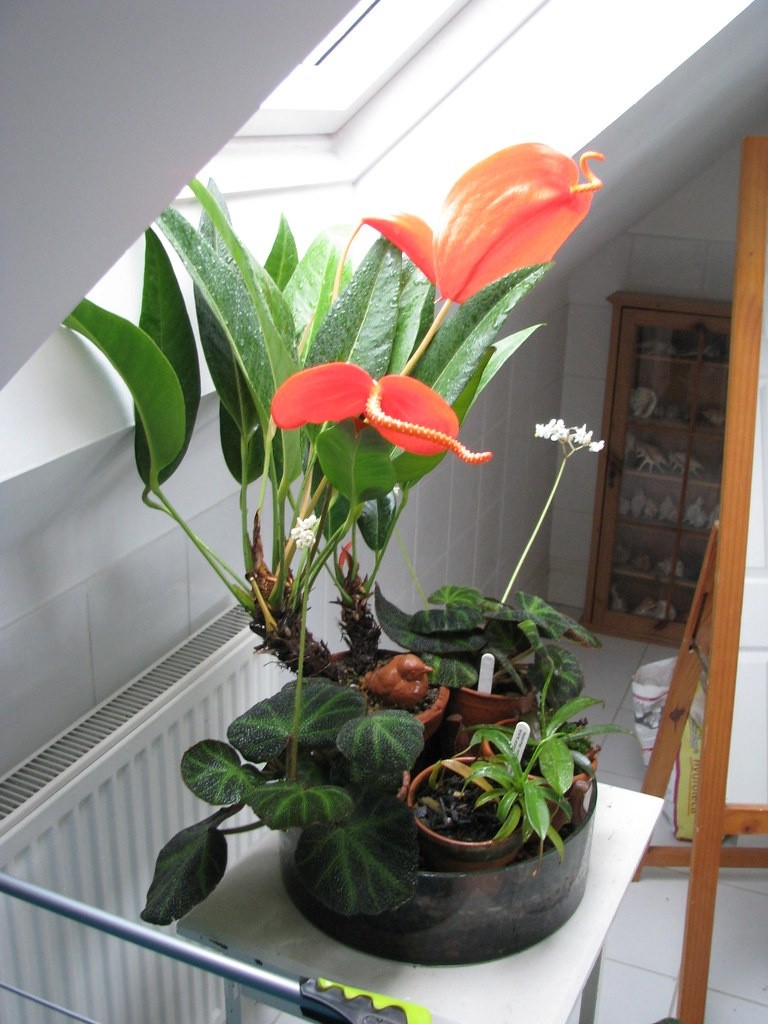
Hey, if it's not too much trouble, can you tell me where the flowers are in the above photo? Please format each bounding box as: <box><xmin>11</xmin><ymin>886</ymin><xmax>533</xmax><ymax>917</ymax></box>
<box><xmin>374</xmin><ymin>416</ymin><xmax>604</xmax><ymax>706</ymax></box>
<box><xmin>61</xmin><ymin>140</ymin><xmax>604</xmax><ymax>709</ymax></box>
<box><xmin>140</xmin><ymin>514</ymin><xmax>425</xmax><ymax>927</ymax></box>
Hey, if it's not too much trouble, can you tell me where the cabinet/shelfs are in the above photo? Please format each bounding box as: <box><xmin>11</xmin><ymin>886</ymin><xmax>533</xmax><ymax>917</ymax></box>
<box><xmin>579</xmin><ymin>292</ymin><xmax>731</xmax><ymax>648</ymax></box>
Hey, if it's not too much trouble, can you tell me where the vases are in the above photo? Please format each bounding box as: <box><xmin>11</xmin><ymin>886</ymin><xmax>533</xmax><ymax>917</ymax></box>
<box><xmin>451</xmin><ymin>664</ymin><xmax>542</xmax><ymax>755</ymax></box>
<box><xmin>330</xmin><ymin>649</ymin><xmax>450</xmax><ymax>777</ymax></box>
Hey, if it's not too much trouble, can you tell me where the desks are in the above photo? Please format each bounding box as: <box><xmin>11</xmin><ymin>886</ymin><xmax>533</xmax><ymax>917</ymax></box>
<box><xmin>175</xmin><ymin>781</ymin><xmax>666</xmax><ymax>1024</ymax></box>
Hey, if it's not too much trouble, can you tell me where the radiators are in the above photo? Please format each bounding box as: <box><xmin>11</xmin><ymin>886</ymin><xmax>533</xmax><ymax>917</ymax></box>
<box><xmin>0</xmin><ymin>602</ymin><xmax>284</xmax><ymax>1024</ymax></box>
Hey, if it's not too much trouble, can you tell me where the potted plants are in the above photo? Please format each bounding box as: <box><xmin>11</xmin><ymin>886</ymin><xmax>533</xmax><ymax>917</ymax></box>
<box><xmin>407</xmin><ymin>669</ymin><xmax>638</xmax><ymax>870</ymax></box>
